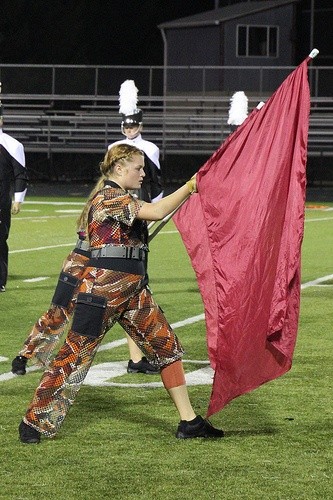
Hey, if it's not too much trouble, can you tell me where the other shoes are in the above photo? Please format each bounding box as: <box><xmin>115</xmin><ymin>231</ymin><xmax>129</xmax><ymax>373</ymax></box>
<box><xmin>18</xmin><ymin>420</ymin><xmax>40</xmax><ymax>444</ymax></box>
<box><xmin>11</xmin><ymin>355</ymin><xmax>28</xmax><ymax>375</ymax></box>
<box><xmin>127</xmin><ymin>356</ymin><xmax>161</xmax><ymax>374</ymax></box>
<box><xmin>176</xmin><ymin>414</ymin><xmax>225</xmax><ymax>439</ymax></box>
<box><xmin>0</xmin><ymin>285</ymin><xmax>6</xmax><ymax>292</ymax></box>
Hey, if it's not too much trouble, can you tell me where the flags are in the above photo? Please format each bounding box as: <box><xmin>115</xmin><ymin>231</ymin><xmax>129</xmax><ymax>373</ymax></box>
<box><xmin>171</xmin><ymin>58</ymin><xmax>311</xmax><ymax>421</ymax></box>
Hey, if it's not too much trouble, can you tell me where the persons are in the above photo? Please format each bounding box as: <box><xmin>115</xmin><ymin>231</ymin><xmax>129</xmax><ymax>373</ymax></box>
<box><xmin>18</xmin><ymin>144</ymin><xmax>225</xmax><ymax>444</ymax></box>
<box><xmin>0</xmin><ymin>102</ymin><xmax>27</xmax><ymax>292</ymax></box>
<box><xmin>11</xmin><ymin>162</ymin><xmax>165</xmax><ymax>375</ymax></box>
<box><xmin>103</xmin><ymin>78</ymin><xmax>163</xmax><ymax>206</ymax></box>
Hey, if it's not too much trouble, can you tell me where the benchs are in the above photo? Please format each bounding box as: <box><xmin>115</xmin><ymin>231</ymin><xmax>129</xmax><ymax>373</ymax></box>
<box><xmin>0</xmin><ymin>93</ymin><xmax>333</xmax><ymax>157</ymax></box>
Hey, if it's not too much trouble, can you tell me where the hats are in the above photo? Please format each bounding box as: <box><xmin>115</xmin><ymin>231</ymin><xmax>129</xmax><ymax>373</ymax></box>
<box><xmin>227</xmin><ymin>91</ymin><xmax>249</xmax><ymax>131</ymax></box>
<box><xmin>118</xmin><ymin>80</ymin><xmax>143</xmax><ymax>126</ymax></box>
